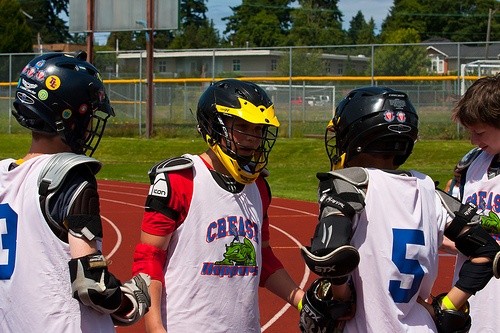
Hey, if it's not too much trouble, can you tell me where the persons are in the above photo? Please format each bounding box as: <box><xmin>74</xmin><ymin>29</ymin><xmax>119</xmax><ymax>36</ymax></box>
<box><xmin>299</xmin><ymin>86</ymin><xmax>500</xmax><ymax>333</ymax></box>
<box><xmin>444</xmin><ymin>169</ymin><xmax>461</xmax><ymax>200</ymax></box>
<box><xmin>453</xmin><ymin>73</ymin><xmax>500</xmax><ymax>333</ymax></box>
<box><xmin>0</xmin><ymin>51</ymin><xmax>151</xmax><ymax>333</ymax></box>
<box><xmin>131</xmin><ymin>79</ymin><xmax>305</xmax><ymax>333</ymax></box>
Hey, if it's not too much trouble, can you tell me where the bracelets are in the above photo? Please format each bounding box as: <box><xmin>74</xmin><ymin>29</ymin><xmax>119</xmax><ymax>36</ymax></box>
<box><xmin>442</xmin><ymin>295</ymin><xmax>456</xmax><ymax>310</ymax></box>
<box><xmin>298</xmin><ymin>300</ymin><xmax>302</xmax><ymax>311</ymax></box>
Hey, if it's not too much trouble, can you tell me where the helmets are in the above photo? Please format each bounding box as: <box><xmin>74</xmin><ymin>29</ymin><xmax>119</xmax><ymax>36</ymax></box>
<box><xmin>196</xmin><ymin>79</ymin><xmax>281</xmax><ymax>185</ymax></box>
<box><xmin>325</xmin><ymin>87</ymin><xmax>419</xmax><ymax>171</ymax></box>
<box><xmin>11</xmin><ymin>50</ymin><xmax>116</xmax><ymax>158</ymax></box>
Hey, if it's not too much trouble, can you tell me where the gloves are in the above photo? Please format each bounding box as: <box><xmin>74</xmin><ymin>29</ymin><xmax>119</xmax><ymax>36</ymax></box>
<box><xmin>299</xmin><ymin>275</ymin><xmax>356</xmax><ymax>333</ymax></box>
<box><xmin>109</xmin><ymin>273</ymin><xmax>151</xmax><ymax>326</ymax></box>
<box><xmin>432</xmin><ymin>292</ymin><xmax>471</xmax><ymax>333</ymax></box>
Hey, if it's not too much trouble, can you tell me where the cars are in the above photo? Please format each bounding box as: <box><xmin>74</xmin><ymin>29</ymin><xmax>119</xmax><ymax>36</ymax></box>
<box><xmin>290</xmin><ymin>94</ymin><xmax>329</xmax><ymax>107</ymax></box>
